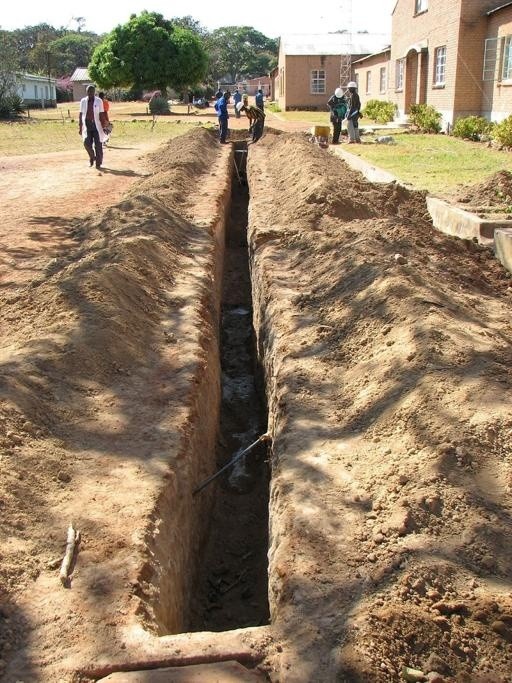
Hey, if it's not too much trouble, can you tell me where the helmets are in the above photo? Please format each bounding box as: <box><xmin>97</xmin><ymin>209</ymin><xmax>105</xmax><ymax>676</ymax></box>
<box><xmin>346</xmin><ymin>81</ymin><xmax>357</xmax><ymax>87</ymax></box>
<box><xmin>235</xmin><ymin>101</ymin><xmax>244</xmax><ymax>111</ymax></box>
<box><xmin>335</xmin><ymin>87</ymin><xmax>344</xmax><ymax>99</ymax></box>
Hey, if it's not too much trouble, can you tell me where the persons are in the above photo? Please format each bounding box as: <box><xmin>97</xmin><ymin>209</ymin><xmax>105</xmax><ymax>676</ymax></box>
<box><xmin>194</xmin><ymin>86</ymin><xmax>264</xmax><ymax>117</ymax></box>
<box><xmin>79</xmin><ymin>85</ymin><xmax>106</xmax><ymax>168</ymax></box>
<box><xmin>236</xmin><ymin>102</ymin><xmax>266</xmax><ymax>143</ymax></box>
<box><xmin>98</xmin><ymin>92</ymin><xmax>110</xmax><ymax>146</ymax></box>
<box><xmin>214</xmin><ymin>92</ymin><xmax>231</xmax><ymax>144</ymax></box>
<box><xmin>344</xmin><ymin>82</ymin><xmax>360</xmax><ymax>144</ymax></box>
<box><xmin>327</xmin><ymin>88</ymin><xmax>348</xmax><ymax>144</ymax></box>
